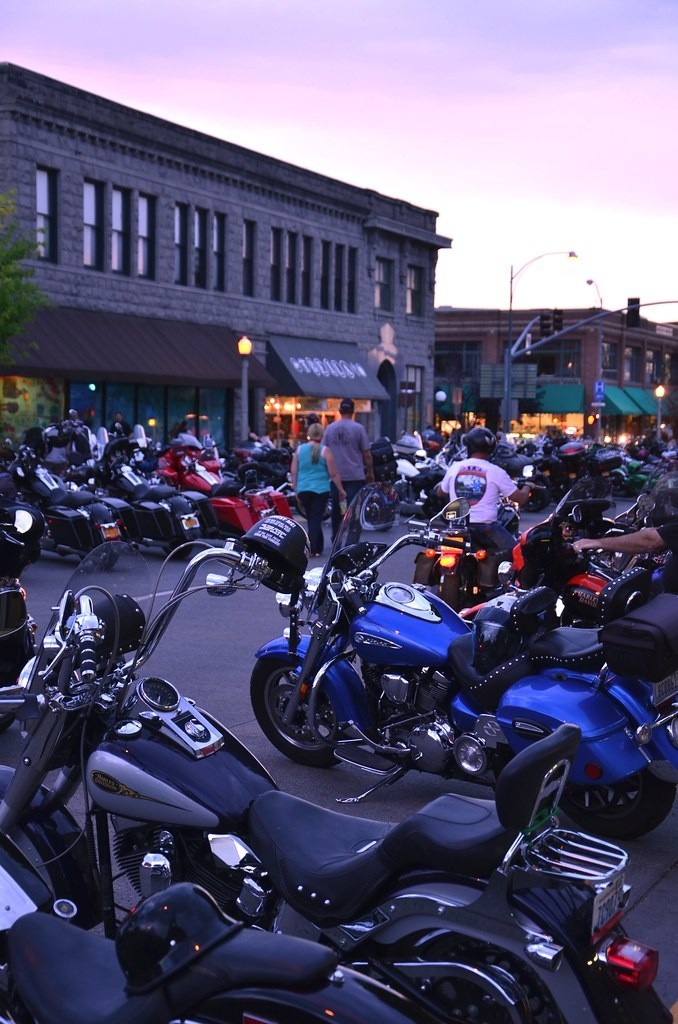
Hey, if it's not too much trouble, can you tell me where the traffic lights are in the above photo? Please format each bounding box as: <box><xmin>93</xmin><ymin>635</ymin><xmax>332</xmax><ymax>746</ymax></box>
<box><xmin>540</xmin><ymin>314</ymin><xmax>552</xmax><ymax>337</ymax></box>
<box><xmin>553</xmin><ymin>309</ymin><xmax>563</xmax><ymax>333</ymax></box>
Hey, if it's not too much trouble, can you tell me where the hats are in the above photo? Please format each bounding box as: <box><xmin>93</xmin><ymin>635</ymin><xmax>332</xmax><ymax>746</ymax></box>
<box><xmin>339</xmin><ymin>399</ymin><xmax>355</xmax><ymax>413</ymax></box>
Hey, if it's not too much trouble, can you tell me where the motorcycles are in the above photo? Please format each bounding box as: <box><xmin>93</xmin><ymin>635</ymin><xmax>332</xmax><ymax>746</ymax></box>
<box><xmin>1</xmin><ymin>538</ymin><xmax>678</xmax><ymax>1024</ymax></box>
<box><xmin>1</xmin><ymin>845</ymin><xmax>416</xmax><ymax>1024</ymax></box>
<box><xmin>0</xmin><ymin>409</ymin><xmax>678</xmax><ymax>629</ymax></box>
<box><xmin>250</xmin><ymin>480</ymin><xmax>678</xmax><ymax>841</ymax></box>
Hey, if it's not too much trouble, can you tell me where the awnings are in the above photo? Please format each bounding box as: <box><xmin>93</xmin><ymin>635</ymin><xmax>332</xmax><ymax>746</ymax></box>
<box><xmin>602</xmin><ymin>385</ymin><xmax>674</xmax><ymax>415</ymax></box>
<box><xmin>266</xmin><ymin>336</ymin><xmax>391</xmax><ymax>400</ymax></box>
<box><xmin>432</xmin><ymin>382</ymin><xmax>585</xmax><ymax>415</ymax></box>
<box><xmin>0</xmin><ymin>304</ymin><xmax>280</xmax><ymax>389</ymax></box>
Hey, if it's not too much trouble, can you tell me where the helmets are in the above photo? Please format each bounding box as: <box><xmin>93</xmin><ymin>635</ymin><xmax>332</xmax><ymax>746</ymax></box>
<box><xmin>243</xmin><ymin>514</ymin><xmax>311</xmax><ymax>577</ymax></box>
<box><xmin>306</xmin><ymin>413</ymin><xmax>319</xmax><ymax>419</ymax></box>
<box><xmin>42</xmin><ymin>426</ymin><xmax>77</xmax><ymax>447</ymax></box>
<box><xmin>112</xmin><ymin>881</ymin><xmax>244</xmax><ymax>999</ymax></box>
<box><xmin>134</xmin><ymin>456</ymin><xmax>158</xmax><ymax>474</ymax></box>
<box><xmin>464</xmin><ymin>427</ymin><xmax>496</xmax><ymax>454</ymax></box>
<box><xmin>474</xmin><ymin>595</ymin><xmax>523</xmax><ymax>674</ymax></box>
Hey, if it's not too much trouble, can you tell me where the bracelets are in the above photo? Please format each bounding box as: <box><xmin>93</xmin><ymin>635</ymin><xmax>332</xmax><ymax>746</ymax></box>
<box><xmin>524</xmin><ymin>484</ymin><xmax>533</xmax><ymax>491</ymax></box>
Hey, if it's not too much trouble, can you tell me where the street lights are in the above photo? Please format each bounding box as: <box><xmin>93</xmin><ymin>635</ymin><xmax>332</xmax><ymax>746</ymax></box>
<box><xmin>238</xmin><ymin>336</ymin><xmax>252</xmax><ymax>441</ymax></box>
<box><xmin>655</xmin><ymin>385</ymin><xmax>665</xmax><ymax>438</ymax></box>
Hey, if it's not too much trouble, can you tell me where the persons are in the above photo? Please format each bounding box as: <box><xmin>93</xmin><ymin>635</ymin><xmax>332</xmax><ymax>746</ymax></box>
<box><xmin>248</xmin><ymin>431</ymin><xmax>276</xmax><ymax>449</ymax></box>
<box><xmin>175</xmin><ymin>420</ymin><xmax>192</xmax><ymax>439</ymax></box>
<box><xmin>291</xmin><ymin>423</ymin><xmax>347</xmax><ymax>556</ymax></box>
<box><xmin>320</xmin><ymin>399</ymin><xmax>374</xmax><ymax>551</ymax></box>
<box><xmin>438</xmin><ymin>427</ymin><xmax>535</xmax><ymax>564</ymax></box>
<box><xmin>109</xmin><ymin>411</ymin><xmax>135</xmax><ymax>463</ymax></box>
<box><xmin>422</xmin><ymin>425</ymin><xmax>435</xmax><ymax>441</ymax></box>
<box><xmin>573</xmin><ymin>519</ymin><xmax>678</xmax><ymax>595</ymax></box>
<box><xmin>66</xmin><ymin>409</ymin><xmax>84</xmax><ymax>428</ymax></box>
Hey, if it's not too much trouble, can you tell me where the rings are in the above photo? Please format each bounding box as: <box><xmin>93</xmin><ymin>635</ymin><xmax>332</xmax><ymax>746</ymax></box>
<box><xmin>576</xmin><ymin>546</ymin><xmax>578</xmax><ymax>548</ymax></box>
